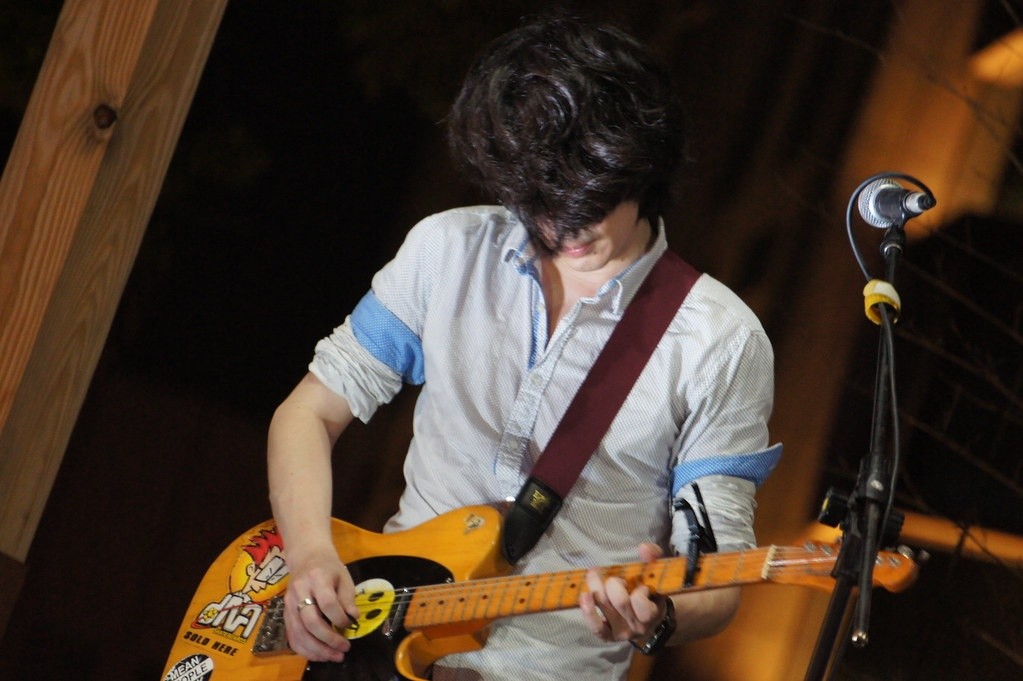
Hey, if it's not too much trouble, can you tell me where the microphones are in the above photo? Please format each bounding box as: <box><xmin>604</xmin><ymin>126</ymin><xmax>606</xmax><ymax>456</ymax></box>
<box><xmin>858</xmin><ymin>178</ymin><xmax>936</xmax><ymax>229</ymax></box>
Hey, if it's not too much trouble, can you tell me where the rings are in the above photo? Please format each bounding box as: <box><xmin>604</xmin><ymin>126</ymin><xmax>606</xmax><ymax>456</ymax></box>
<box><xmin>296</xmin><ymin>599</ymin><xmax>315</xmax><ymax>611</ymax></box>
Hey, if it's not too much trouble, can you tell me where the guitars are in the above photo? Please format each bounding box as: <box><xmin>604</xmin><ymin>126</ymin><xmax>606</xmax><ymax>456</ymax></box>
<box><xmin>156</xmin><ymin>503</ymin><xmax>930</xmax><ymax>681</ymax></box>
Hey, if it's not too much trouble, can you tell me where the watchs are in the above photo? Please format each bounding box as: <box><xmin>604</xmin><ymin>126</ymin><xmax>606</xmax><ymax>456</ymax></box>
<box><xmin>628</xmin><ymin>596</ymin><xmax>677</xmax><ymax>656</ymax></box>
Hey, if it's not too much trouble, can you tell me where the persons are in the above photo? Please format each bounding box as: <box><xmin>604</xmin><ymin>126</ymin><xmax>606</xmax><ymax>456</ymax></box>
<box><xmin>269</xmin><ymin>17</ymin><xmax>783</xmax><ymax>681</ymax></box>
